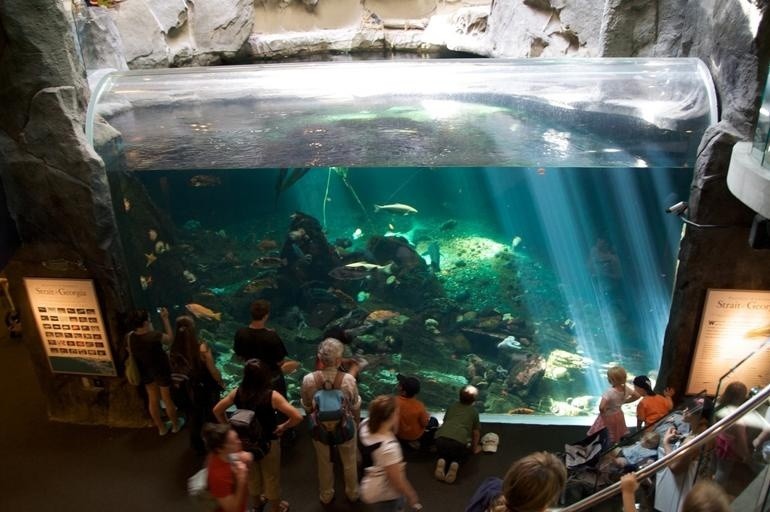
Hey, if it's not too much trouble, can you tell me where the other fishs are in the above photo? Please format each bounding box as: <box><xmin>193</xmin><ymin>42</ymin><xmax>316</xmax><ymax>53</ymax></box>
<box><xmin>112</xmin><ymin>166</ymin><xmax>616</xmax><ymax>415</ymax></box>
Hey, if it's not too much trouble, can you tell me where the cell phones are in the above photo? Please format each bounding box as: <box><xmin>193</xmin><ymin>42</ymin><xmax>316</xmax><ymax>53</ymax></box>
<box><xmin>156</xmin><ymin>307</ymin><xmax>162</xmax><ymax>314</ymax></box>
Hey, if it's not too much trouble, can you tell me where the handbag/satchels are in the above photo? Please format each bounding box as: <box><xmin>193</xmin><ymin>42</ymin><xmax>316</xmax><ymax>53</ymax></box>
<box><xmin>125</xmin><ymin>355</ymin><xmax>141</xmax><ymax>386</ymax></box>
<box><xmin>359</xmin><ymin>462</ymin><xmax>406</xmax><ymax>504</ymax></box>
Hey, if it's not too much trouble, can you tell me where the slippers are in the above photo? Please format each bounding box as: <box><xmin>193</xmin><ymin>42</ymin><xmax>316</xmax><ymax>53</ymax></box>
<box><xmin>172</xmin><ymin>417</ymin><xmax>185</xmax><ymax>433</ymax></box>
<box><xmin>159</xmin><ymin>420</ymin><xmax>173</xmax><ymax>435</ymax></box>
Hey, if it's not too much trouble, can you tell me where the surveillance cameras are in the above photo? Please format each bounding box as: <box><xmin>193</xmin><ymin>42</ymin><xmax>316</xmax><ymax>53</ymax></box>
<box><xmin>664</xmin><ymin>201</ymin><xmax>688</xmax><ymax>216</ymax></box>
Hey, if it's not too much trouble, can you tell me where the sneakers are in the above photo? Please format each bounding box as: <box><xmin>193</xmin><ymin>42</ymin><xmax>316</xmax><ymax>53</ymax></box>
<box><xmin>251</xmin><ymin>494</ymin><xmax>268</xmax><ymax>511</ymax></box>
<box><xmin>319</xmin><ymin>488</ymin><xmax>335</xmax><ymax>504</ymax></box>
<box><xmin>345</xmin><ymin>486</ymin><xmax>360</xmax><ymax>501</ymax></box>
<box><xmin>279</xmin><ymin>500</ymin><xmax>288</xmax><ymax>512</ymax></box>
<box><xmin>435</xmin><ymin>458</ymin><xmax>445</xmax><ymax>481</ymax></box>
<box><xmin>445</xmin><ymin>462</ymin><xmax>459</xmax><ymax>483</ymax></box>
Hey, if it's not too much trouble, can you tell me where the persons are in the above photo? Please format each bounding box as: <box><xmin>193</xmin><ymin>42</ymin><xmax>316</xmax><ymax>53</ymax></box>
<box><xmin>124</xmin><ymin>299</ymin><xmax>770</xmax><ymax>511</ymax></box>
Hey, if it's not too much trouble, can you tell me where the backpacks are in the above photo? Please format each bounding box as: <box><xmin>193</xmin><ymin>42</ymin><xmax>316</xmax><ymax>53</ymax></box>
<box><xmin>169</xmin><ymin>342</ymin><xmax>200</xmax><ymax>413</ymax></box>
<box><xmin>305</xmin><ymin>371</ymin><xmax>355</xmax><ymax>448</ymax></box>
<box><xmin>225</xmin><ymin>388</ymin><xmax>272</xmax><ymax>444</ymax></box>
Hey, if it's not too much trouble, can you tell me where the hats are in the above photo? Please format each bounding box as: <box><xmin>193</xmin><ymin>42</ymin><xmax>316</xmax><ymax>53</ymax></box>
<box><xmin>481</xmin><ymin>432</ymin><xmax>499</xmax><ymax>452</ymax></box>
<box><xmin>395</xmin><ymin>373</ymin><xmax>420</xmax><ymax>394</ymax></box>
